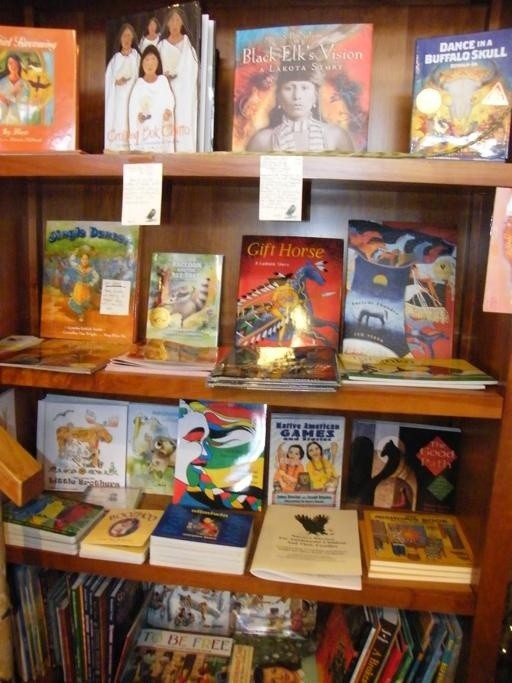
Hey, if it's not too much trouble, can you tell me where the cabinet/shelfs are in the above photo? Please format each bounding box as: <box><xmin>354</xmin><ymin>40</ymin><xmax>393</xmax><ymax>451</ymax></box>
<box><xmin>1</xmin><ymin>1</ymin><xmax>512</xmax><ymax>683</ymax></box>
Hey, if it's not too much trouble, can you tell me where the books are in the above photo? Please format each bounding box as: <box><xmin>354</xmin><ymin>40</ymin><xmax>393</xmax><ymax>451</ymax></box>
<box><xmin>1</xmin><ymin>388</ymin><xmax>482</xmax><ymax>591</ymax></box>
<box><xmin>15</xmin><ymin>565</ymin><xmax>462</xmax><ymax>682</ymax></box>
<box><xmin>1</xmin><ymin>220</ymin><xmax>497</xmax><ymax>392</ymax></box>
<box><xmin>0</xmin><ymin>1</ymin><xmax>511</xmax><ymax>162</ymax></box>
